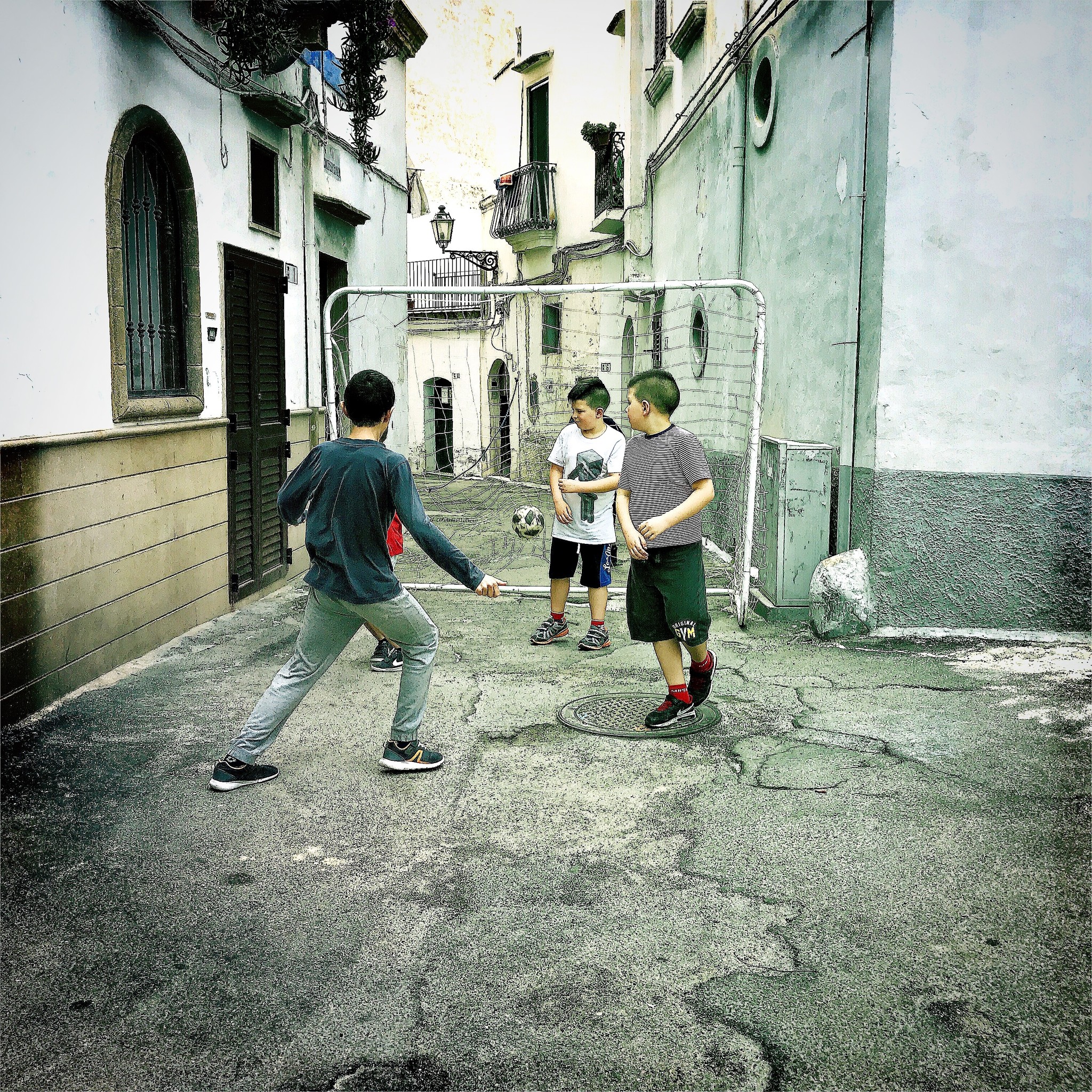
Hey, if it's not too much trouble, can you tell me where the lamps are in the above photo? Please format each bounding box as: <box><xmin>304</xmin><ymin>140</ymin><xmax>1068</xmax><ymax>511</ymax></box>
<box><xmin>429</xmin><ymin>206</ymin><xmax>498</xmax><ymax>271</ymax></box>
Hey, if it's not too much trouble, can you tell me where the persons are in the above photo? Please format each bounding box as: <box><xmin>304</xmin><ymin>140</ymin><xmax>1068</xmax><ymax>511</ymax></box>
<box><xmin>566</xmin><ymin>414</ymin><xmax>626</xmax><ymax>565</ymax></box>
<box><xmin>616</xmin><ymin>370</ymin><xmax>718</xmax><ymax>728</ymax></box>
<box><xmin>531</xmin><ymin>378</ymin><xmax>626</xmax><ymax>650</ymax></box>
<box><xmin>364</xmin><ymin>425</ymin><xmax>404</xmax><ymax>672</ymax></box>
<box><xmin>210</xmin><ymin>369</ymin><xmax>508</xmax><ymax>793</ymax></box>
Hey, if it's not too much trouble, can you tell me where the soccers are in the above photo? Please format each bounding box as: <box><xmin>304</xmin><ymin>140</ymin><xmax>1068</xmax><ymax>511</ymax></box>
<box><xmin>512</xmin><ymin>506</ymin><xmax>544</xmax><ymax>540</ymax></box>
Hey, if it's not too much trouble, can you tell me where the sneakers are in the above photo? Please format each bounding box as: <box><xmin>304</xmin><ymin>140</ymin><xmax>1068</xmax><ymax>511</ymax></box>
<box><xmin>645</xmin><ymin>694</ymin><xmax>696</xmax><ymax>728</ymax></box>
<box><xmin>577</xmin><ymin>625</ymin><xmax>610</xmax><ymax>650</ymax></box>
<box><xmin>370</xmin><ymin>647</ymin><xmax>404</xmax><ymax>671</ymax></box>
<box><xmin>209</xmin><ymin>759</ymin><xmax>279</xmax><ymax>790</ymax></box>
<box><xmin>371</xmin><ymin>640</ymin><xmax>394</xmax><ymax>661</ymax></box>
<box><xmin>378</xmin><ymin>739</ymin><xmax>445</xmax><ymax>770</ymax></box>
<box><xmin>687</xmin><ymin>650</ymin><xmax>717</xmax><ymax>707</ymax></box>
<box><xmin>529</xmin><ymin>615</ymin><xmax>569</xmax><ymax>644</ymax></box>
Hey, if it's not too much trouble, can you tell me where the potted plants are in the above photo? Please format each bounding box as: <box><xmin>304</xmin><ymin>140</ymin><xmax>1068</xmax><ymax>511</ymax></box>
<box><xmin>581</xmin><ymin>121</ymin><xmax>617</xmax><ymax>152</ymax></box>
